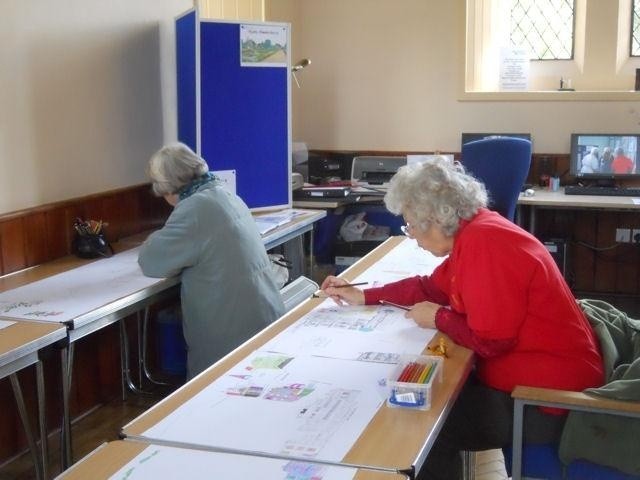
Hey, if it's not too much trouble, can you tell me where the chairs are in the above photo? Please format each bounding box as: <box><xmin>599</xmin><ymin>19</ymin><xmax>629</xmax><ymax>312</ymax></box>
<box><xmin>392</xmin><ymin>138</ymin><xmax>534</xmax><ymax>237</ymax></box>
<box><xmin>509</xmin><ymin>386</ymin><xmax>639</xmax><ymax>480</ymax></box>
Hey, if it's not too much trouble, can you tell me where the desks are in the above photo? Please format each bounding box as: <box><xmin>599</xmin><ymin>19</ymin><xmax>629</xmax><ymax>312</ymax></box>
<box><xmin>293</xmin><ymin>186</ymin><xmax>524</xmax><ymax>229</ymax></box>
<box><xmin>516</xmin><ymin>186</ymin><xmax>639</xmax><ymax>237</ymax></box>
<box><xmin>0</xmin><ymin>207</ymin><xmax>480</xmax><ymax>480</ymax></box>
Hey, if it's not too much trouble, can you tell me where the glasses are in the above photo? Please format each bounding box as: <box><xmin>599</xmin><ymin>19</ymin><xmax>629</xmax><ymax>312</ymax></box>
<box><xmin>401</xmin><ymin>221</ymin><xmax>419</xmax><ymax>238</ymax></box>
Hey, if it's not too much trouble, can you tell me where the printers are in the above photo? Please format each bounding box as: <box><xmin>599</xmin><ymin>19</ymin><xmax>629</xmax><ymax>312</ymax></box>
<box><xmin>352</xmin><ymin>156</ymin><xmax>407</xmax><ymax>196</ymax></box>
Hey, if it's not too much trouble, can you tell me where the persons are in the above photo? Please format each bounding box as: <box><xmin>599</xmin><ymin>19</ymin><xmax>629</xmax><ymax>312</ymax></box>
<box><xmin>320</xmin><ymin>152</ymin><xmax>605</xmax><ymax>479</ymax></box>
<box><xmin>599</xmin><ymin>147</ymin><xmax>615</xmax><ymax>173</ymax></box>
<box><xmin>610</xmin><ymin>147</ymin><xmax>634</xmax><ymax>174</ymax></box>
<box><xmin>580</xmin><ymin>147</ymin><xmax>600</xmax><ymax>174</ymax></box>
<box><xmin>138</xmin><ymin>141</ymin><xmax>287</xmax><ymax>386</ymax></box>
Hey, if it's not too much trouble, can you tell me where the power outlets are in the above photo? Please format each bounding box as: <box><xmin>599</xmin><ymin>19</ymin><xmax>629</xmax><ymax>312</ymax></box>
<box><xmin>633</xmin><ymin>229</ymin><xmax>640</xmax><ymax>243</ymax></box>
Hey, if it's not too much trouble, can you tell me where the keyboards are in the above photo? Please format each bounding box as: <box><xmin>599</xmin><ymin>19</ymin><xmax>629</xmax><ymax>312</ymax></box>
<box><xmin>520</xmin><ymin>183</ymin><xmax>532</xmax><ymax>191</ymax></box>
<box><xmin>565</xmin><ymin>187</ymin><xmax>640</xmax><ymax>196</ymax></box>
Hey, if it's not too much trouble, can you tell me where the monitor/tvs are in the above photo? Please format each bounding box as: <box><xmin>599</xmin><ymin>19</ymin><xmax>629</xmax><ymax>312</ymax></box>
<box><xmin>570</xmin><ymin>133</ymin><xmax>640</xmax><ymax>186</ymax></box>
<box><xmin>462</xmin><ymin>132</ymin><xmax>531</xmax><ymax>174</ymax></box>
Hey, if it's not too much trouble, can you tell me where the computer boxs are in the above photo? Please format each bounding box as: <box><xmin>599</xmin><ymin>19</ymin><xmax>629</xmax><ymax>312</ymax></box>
<box><xmin>537</xmin><ymin>231</ymin><xmax>575</xmax><ymax>298</ymax></box>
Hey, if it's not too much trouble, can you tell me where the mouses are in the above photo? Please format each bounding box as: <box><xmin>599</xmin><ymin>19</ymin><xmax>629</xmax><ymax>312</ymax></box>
<box><xmin>524</xmin><ymin>189</ymin><xmax>534</xmax><ymax>197</ymax></box>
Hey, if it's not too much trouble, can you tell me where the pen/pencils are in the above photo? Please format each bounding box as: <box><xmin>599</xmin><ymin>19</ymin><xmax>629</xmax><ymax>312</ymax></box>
<box><xmin>320</xmin><ymin>282</ymin><xmax>368</xmax><ymax>291</ymax></box>
<box><xmin>379</xmin><ymin>299</ymin><xmax>411</xmax><ymax>311</ymax></box>
<box><xmin>397</xmin><ymin>362</ymin><xmax>437</xmax><ymax>384</ymax></box>
<box><xmin>71</xmin><ymin>216</ymin><xmax>109</xmax><ymax>236</ymax></box>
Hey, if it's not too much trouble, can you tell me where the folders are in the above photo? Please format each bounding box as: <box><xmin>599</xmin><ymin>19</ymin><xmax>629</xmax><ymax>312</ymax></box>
<box><xmin>296</xmin><ymin>186</ymin><xmax>352</xmax><ymax>198</ymax></box>
